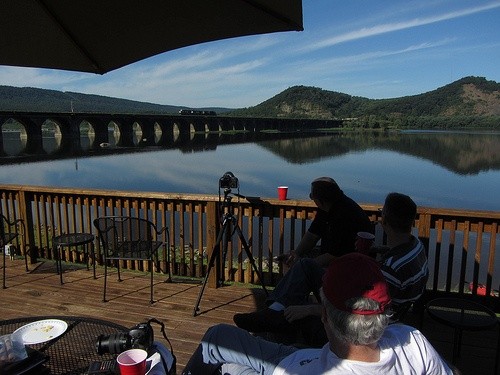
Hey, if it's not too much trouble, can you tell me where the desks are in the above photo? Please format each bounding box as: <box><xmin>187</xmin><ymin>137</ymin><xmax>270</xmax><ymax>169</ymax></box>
<box><xmin>0</xmin><ymin>315</ymin><xmax>129</xmax><ymax>375</ymax></box>
<box><xmin>52</xmin><ymin>233</ymin><xmax>96</xmax><ymax>284</ymax></box>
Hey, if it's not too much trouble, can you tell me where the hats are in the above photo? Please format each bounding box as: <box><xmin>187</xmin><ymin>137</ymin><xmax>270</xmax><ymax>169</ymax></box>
<box><xmin>322</xmin><ymin>252</ymin><xmax>393</xmax><ymax>315</ymax></box>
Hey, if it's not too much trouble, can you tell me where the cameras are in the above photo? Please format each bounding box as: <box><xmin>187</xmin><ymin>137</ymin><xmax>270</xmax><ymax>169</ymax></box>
<box><xmin>96</xmin><ymin>321</ymin><xmax>154</xmax><ymax>355</ymax></box>
<box><xmin>220</xmin><ymin>172</ymin><xmax>237</xmax><ymax>214</ymax></box>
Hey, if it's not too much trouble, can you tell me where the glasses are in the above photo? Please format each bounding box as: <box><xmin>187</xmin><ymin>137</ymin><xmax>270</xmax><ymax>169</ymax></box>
<box><xmin>378</xmin><ymin>208</ymin><xmax>384</xmax><ymax>214</ymax></box>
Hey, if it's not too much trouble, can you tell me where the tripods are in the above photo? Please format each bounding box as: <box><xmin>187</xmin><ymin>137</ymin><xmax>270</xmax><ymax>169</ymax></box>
<box><xmin>193</xmin><ymin>214</ymin><xmax>270</xmax><ymax>316</ymax></box>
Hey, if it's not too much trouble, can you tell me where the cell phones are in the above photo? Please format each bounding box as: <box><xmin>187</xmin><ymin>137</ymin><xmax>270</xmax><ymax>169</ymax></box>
<box><xmin>88</xmin><ymin>360</ymin><xmax>117</xmax><ymax>375</ymax></box>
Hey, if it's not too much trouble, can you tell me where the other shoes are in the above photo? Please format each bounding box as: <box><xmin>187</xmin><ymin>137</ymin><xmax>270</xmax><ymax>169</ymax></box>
<box><xmin>233</xmin><ymin>307</ymin><xmax>292</xmax><ymax>335</ymax></box>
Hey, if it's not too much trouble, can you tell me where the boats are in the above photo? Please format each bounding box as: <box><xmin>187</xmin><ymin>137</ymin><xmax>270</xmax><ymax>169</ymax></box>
<box><xmin>469</xmin><ymin>282</ymin><xmax>500</xmax><ymax>297</ymax></box>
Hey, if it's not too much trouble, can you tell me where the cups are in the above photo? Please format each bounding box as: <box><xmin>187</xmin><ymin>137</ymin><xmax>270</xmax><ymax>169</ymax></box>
<box><xmin>116</xmin><ymin>349</ymin><xmax>148</xmax><ymax>375</ymax></box>
<box><xmin>357</xmin><ymin>232</ymin><xmax>375</xmax><ymax>254</ymax></box>
<box><xmin>277</xmin><ymin>187</ymin><xmax>288</xmax><ymax>200</ymax></box>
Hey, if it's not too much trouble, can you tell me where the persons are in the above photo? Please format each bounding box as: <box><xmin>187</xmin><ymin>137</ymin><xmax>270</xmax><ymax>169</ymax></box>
<box><xmin>233</xmin><ymin>191</ymin><xmax>430</xmax><ymax>346</ymax></box>
<box><xmin>274</xmin><ymin>178</ymin><xmax>375</xmax><ymax>267</ymax></box>
<box><xmin>185</xmin><ymin>252</ymin><xmax>460</xmax><ymax>375</ymax></box>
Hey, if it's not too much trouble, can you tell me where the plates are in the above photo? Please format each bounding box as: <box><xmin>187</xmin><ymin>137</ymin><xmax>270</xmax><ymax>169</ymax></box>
<box><xmin>11</xmin><ymin>318</ymin><xmax>68</xmax><ymax>345</ymax></box>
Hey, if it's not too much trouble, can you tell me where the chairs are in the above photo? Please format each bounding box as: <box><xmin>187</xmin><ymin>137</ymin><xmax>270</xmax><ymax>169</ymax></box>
<box><xmin>0</xmin><ymin>213</ymin><xmax>29</xmax><ymax>289</ymax></box>
<box><xmin>93</xmin><ymin>215</ymin><xmax>171</xmax><ymax>304</ymax></box>
<box><xmin>404</xmin><ymin>282</ymin><xmax>500</xmax><ymax>375</ymax></box>
